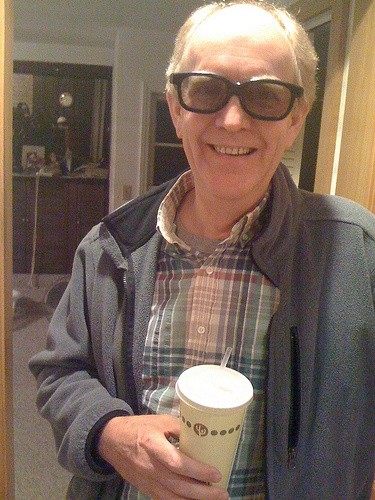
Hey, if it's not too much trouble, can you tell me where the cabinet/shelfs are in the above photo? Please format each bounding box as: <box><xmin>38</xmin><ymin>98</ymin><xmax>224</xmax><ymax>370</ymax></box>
<box><xmin>13</xmin><ymin>176</ymin><xmax>109</xmax><ymax>273</ymax></box>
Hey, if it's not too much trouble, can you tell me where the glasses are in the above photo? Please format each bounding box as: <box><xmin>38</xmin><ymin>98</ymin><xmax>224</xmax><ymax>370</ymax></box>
<box><xmin>169</xmin><ymin>73</ymin><xmax>304</xmax><ymax>121</ymax></box>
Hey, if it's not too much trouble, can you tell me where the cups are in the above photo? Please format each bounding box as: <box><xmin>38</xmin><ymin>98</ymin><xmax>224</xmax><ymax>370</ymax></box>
<box><xmin>176</xmin><ymin>365</ymin><xmax>253</xmax><ymax>491</ymax></box>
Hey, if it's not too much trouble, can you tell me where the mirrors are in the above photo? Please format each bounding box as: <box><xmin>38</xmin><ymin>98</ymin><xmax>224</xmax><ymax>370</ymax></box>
<box><xmin>12</xmin><ymin>60</ymin><xmax>113</xmax><ymax>174</ymax></box>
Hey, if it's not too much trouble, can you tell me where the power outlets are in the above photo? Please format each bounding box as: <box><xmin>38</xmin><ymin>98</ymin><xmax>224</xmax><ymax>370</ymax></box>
<box><xmin>124</xmin><ymin>187</ymin><xmax>131</xmax><ymax>201</ymax></box>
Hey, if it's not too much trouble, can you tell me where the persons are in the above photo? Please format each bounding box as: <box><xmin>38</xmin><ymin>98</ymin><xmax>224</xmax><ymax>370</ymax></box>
<box><xmin>27</xmin><ymin>1</ymin><xmax>375</xmax><ymax>500</ymax></box>
